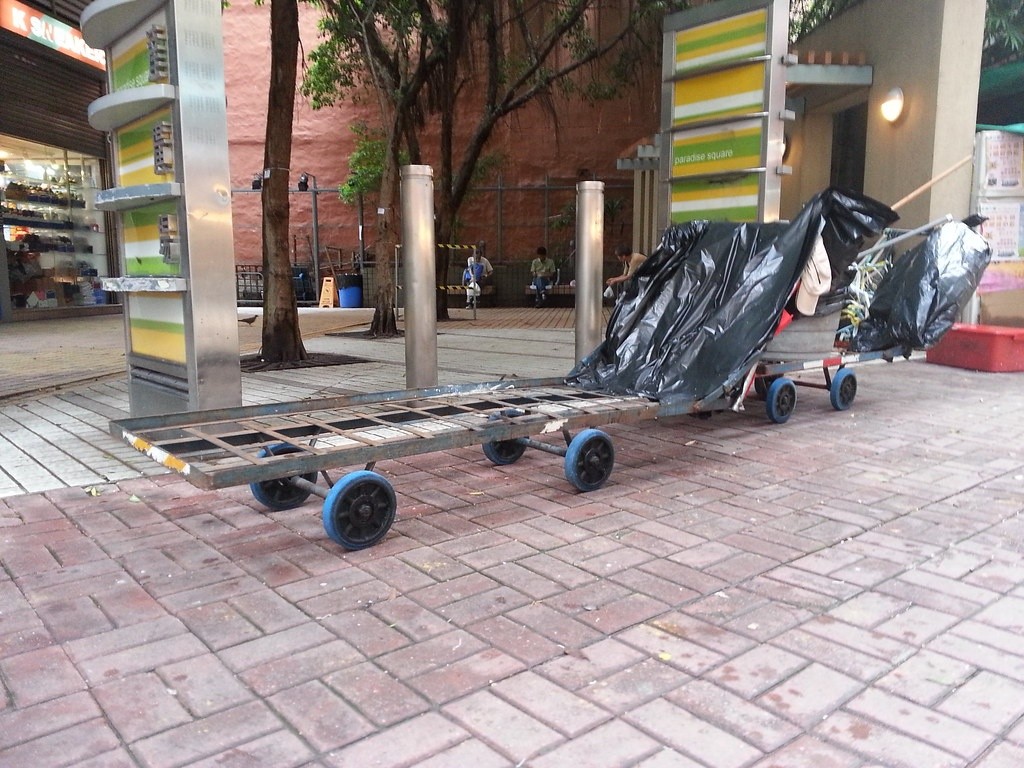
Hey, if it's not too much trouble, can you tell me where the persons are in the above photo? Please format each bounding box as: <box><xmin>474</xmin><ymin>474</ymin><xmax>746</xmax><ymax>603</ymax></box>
<box><xmin>530</xmin><ymin>246</ymin><xmax>556</xmax><ymax>308</ymax></box>
<box><xmin>463</xmin><ymin>249</ymin><xmax>494</xmax><ymax>310</ymax></box>
<box><xmin>605</xmin><ymin>243</ymin><xmax>649</xmax><ymax>290</ymax></box>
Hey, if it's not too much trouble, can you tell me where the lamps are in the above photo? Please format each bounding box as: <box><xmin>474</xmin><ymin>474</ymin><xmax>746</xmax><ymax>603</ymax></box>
<box><xmin>880</xmin><ymin>85</ymin><xmax>904</xmax><ymax>122</ymax></box>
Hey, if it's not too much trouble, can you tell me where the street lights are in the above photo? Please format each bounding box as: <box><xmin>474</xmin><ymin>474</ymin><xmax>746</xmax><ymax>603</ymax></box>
<box><xmin>253</xmin><ymin>169</ymin><xmax>365</xmax><ymax>305</ymax></box>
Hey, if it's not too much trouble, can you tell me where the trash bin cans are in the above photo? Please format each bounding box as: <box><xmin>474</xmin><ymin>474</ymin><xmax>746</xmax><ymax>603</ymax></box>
<box><xmin>334</xmin><ymin>273</ymin><xmax>363</xmax><ymax>308</ymax></box>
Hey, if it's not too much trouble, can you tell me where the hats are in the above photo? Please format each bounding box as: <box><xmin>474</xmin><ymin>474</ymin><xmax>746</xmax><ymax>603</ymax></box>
<box><xmin>474</xmin><ymin>249</ymin><xmax>482</xmax><ymax>259</ymax></box>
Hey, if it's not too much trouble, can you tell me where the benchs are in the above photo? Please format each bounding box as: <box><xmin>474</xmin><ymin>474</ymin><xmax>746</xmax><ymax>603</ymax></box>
<box><xmin>525</xmin><ymin>270</ymin><xmax>575</xmax><ymax>307</ymax></box>
<box><xmin>924</xmin><ymin>322</ymin><xmax>1024</xmax><ymax>372</ymax></box>
<box><xmin>437</xmin><ymin>272</ymin><xmax>498</xmax><ymax>309</ymax></box>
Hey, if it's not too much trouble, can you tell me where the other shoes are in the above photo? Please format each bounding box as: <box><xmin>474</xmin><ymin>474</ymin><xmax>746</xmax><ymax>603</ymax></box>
<box><xmin>465</xmin><ymin>305</ymin><xmax>475</xmax><ymax>310</ymax></box>
<box><xmin>535</xmin><ymin>302</ymin><xmax>545</xmax><ymax>309</ymax></box>
<box><xmin>541</xmin><ymin>292</ymin><xmax>549</xmax><ymax>302</ymax></box>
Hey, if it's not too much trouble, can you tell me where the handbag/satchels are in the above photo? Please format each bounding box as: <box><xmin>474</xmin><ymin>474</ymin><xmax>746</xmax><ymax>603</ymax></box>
<box><xmin>604</xmin><ymin>286</ymin><xmax>616</xmax><ymax>300</ymax></box>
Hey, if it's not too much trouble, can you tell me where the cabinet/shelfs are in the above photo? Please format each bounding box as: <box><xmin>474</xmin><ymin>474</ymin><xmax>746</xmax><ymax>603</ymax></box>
<box><xmin>2</xmin><ymin>172</ymin><xmax>111</xmax><ymax>309</ymax></box>
